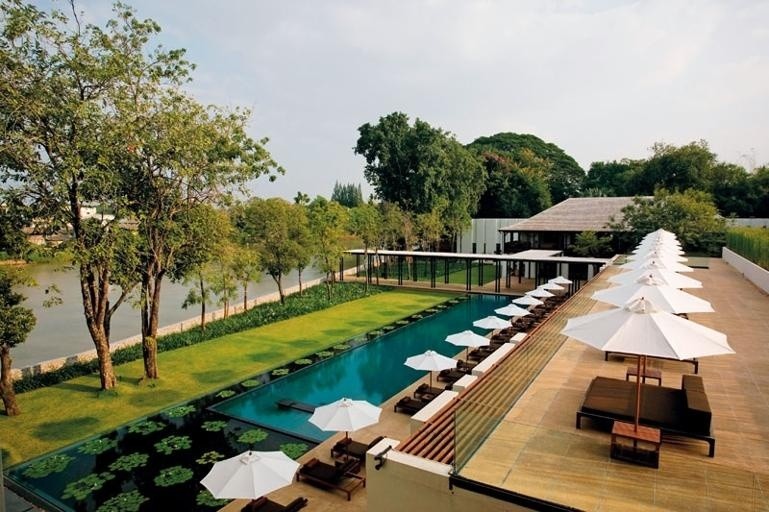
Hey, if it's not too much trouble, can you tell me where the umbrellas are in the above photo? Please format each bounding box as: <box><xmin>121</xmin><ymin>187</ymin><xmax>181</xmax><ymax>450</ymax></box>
<box><xmin>403</xmin><ymin>350</ymin><xmax>458</xmax><ymax>388</ymax></box>
<box><xmin>561</xmin><ymin>227</ymin><xmax>737</xmax><ymax>429</ymax></box>
<box><xmin>511</xmin><ymin>295</ymin><xmax>544</xmax><ymax>306</ymax></box>
<box><xmin>525</xmin><ymin>287</ymin><xmax>557</xmax><ymax>298</ymax></box>
<box><xmin>472</xmin><ymin>316</ymin><xmax>513</xmax><ymax>336</ymax></box>
<box><xmin>548</xmin><ymin>275</ymin><xmax>573</xmax><ymax>285</ymax></box>
<box><xmin>308</xmin><ymin>398</ymin><xmax>383</xmax><ymax>440</ymax></box>
<box><xmin>200</xmin><ymin>450</ymin><xmax>300</xmax><ymax>502</ymax></box>
<box><xmin>537</xmin><ymin>282</ymin><xmax>564</xmax><ymax>290</ymax></box>
<box><xmin>494</xmin><ymin>303</ymin><xmax>534</xmax><ymax>317</ymax></box>
<box><xmin>444</xmin><ymin>329</ymin><xmax>490</xmax><ymax>360</ymax></box>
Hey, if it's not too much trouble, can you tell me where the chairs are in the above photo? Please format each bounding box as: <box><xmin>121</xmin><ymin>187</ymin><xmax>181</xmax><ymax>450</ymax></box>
<box><xmin>576</xmin><ymin>373</ymin><xmax>717</xmax><ymax>458</ymax></box>
<box><xmin>392</xmin><ymin>292</ymin><xmax>571</xmax><ymax>417</ymax></box>
<box><xmin>239</xmin><ymin>435</ymin><xmax>387</xmax><ymax>512</ymax></box>
